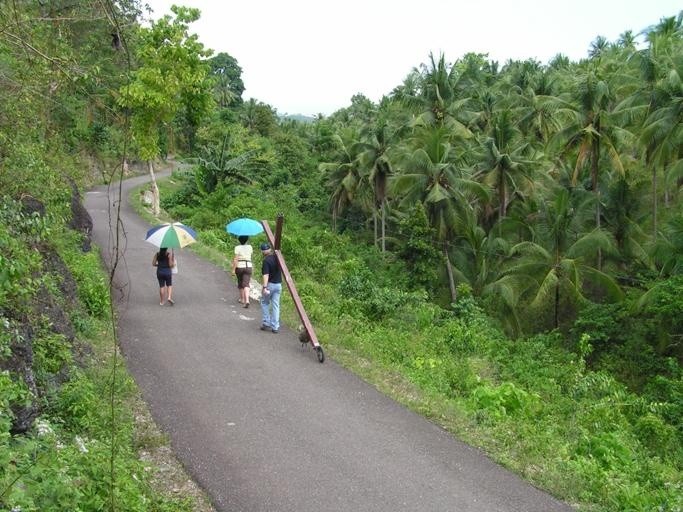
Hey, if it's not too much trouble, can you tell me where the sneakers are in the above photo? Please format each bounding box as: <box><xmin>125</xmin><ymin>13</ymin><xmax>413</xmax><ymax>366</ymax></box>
<box><xmin>261</xmin><ymin>322</ymin><xmax>279</xmax><ymax>333</ymax></box>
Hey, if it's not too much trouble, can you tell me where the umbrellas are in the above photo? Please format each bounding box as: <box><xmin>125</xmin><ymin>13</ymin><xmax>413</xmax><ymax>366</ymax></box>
<box><xmin>226</xmin><ymin>218</ymin><xmax>264</xmax><ymax>245</ymax></box>
<box><xmin>143</xmin><ymin>222</ymin><xmax>197</xmax><ymax>264</ymax></box>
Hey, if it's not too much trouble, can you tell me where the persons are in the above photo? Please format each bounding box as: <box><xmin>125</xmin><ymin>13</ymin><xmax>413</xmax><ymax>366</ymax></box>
<box><xmin>231</xmin><ymin>235</ymin><xmax>254</xmax><ymax>308</ymax></box>
<box><xmin>260</xmin><ymin>244</ymin><xmax>282</xmax><ymax>333</ymax></box>
<box><xmin>153</xmin><ymin>247</ymin><xmax>175</xmax><ymax>305</ymax></box>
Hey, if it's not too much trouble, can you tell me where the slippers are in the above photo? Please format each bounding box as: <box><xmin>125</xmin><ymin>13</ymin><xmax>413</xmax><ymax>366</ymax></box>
<box><xmin>160</xmin><ymin>299</ymin><xmax>174</xmax><ymax>305</ymax></box>
<box><xmin>239</xmin><ymin>299</ymin><xmax>250</xmax><ymax>308</ymax></box>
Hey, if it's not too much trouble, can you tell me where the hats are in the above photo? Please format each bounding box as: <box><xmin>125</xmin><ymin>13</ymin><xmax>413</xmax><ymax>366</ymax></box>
<box><xmin>261</xmin><ymin>243</ymin><xmax>270</xmax><ymax>250</ymax></box>
